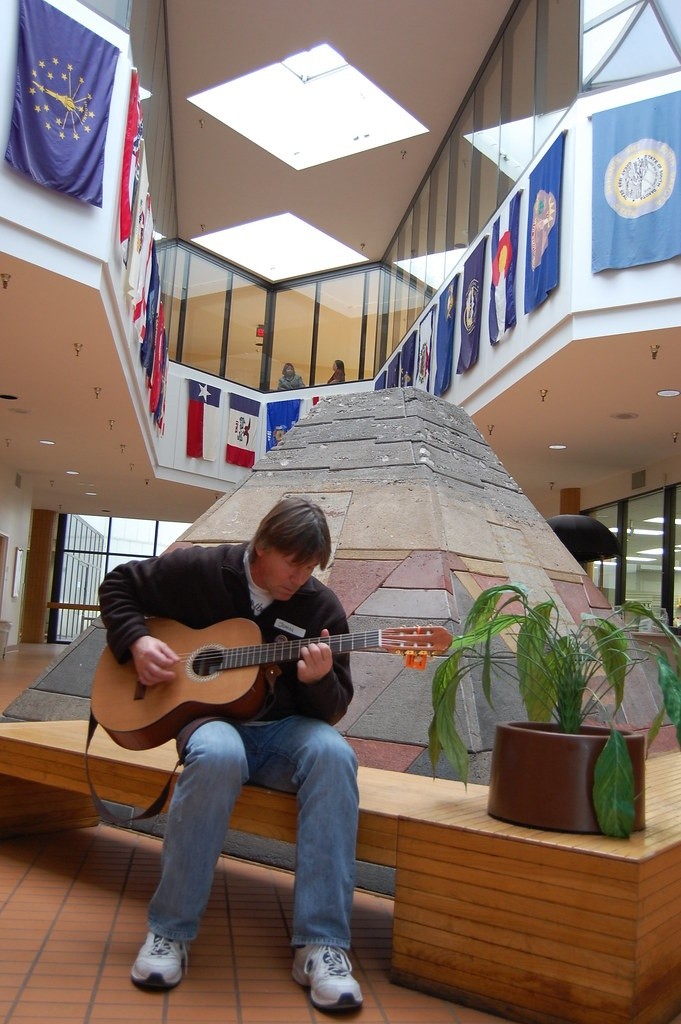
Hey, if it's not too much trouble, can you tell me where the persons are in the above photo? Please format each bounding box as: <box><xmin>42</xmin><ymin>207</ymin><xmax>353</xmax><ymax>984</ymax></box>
<box><xmin>277</xmin><ymin>363</ymin><xmax>305</xmax><ymax>390</ymax></box>
<box><xmin>327</xmin><ymin>360</ymin><xmax>345</xmax><ymax>384</ymax></box>
<box><xmin>98</xmin><ymin>495</ymin><xmax>364</xmax><ymax>1008</ymax></box>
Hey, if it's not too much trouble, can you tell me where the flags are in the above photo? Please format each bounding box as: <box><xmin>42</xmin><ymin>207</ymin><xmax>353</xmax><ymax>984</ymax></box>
<box><xmin>375</xmin><ymin>370</ymin><xmax>387</xmax><ymax>390</ymax></box>
<box><xmin>225</xmin><ymin>393</ymin><xmax>260</xmax><ymax>468</ymax></box>
<box><xmin>120</xmin><ymin>67</ymin><xmax>169</xmax><ymax>437</ymax></box>
<box><xmin>489</xmin><ymin>191</ymin><xmax>521</xmax><ymax>346</ymax></box>
<box><xmin>591</xmin><ymin>90</ymin><xmax>681</xmax><ymax>273</ymax></box>
<box><xmin>456</xmin><ymin>237</ymin><xmax>485</xmax><ymax>374</ymax></box>
<box><xmin>4</xmin><ymin>0</ymin><xmax>121</xmax><ymax>209</ymax></box>
<box><xmin>416</xmin><ymin>305</ymin><xmax>434</xmax><ymax>392</ymax></box>
<box><xmin>261</xmin><ymin>399</ymin><xmax>310</xmax><ymax>459</ymax></box>
<box><xmin>387</xmin><ymin>352</ymin><xmax>399</xmax><ymax>388</ymax></box>
<box><xmin>524</xmin><ymin>132</ymin><xmax>563</xmax><ymax>314</ymax></box>
<box><xmin>400</xmin><ymin>330</ymin><xmax>415</xmax><ymax>387</ymax></box>
<box><xmin>434</xmin><ymin>274</ymin><xmax>458</xmax><ymax>397</ymax></box>
<box><xmin>187</xmin><ymin>379</ymin><xmax>221</xmax><ymax>460</ymax></box>
<box><xmin>313</xmin><ymin>396</ymin><xmax>321</xmax><ymax>407</ymax></box>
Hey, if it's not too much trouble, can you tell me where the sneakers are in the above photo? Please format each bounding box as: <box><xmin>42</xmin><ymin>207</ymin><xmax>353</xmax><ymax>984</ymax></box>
<box><xmin>291</xmin><ymin>942</ymin><xmax>364</xmax><ymax>1009</ymax></box>
<box><xmin>131</xmin><ymin>923</ymin><xmax>189</xmax><ymax>991</ymax></box>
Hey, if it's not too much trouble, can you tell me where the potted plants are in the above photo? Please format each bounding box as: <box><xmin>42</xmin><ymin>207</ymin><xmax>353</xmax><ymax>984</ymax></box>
<box><xmin>428</xmin><ymin>583</ymin><xmax>681</xmax><ymax>840</ymax></box>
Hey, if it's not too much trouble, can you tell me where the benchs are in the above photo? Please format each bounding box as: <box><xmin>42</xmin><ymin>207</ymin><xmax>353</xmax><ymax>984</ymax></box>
<box><xmin>0</xmin><ymin>719</ymin><xmax>681</xmax><ymax>1024</ymax></box>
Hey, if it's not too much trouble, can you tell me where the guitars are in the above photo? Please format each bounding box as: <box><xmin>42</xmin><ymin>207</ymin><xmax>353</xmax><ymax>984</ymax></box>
<box><xmin>89</xmin><ymin>619</ymin><xmax>455</xmax><ymax>754</ymax></box>
